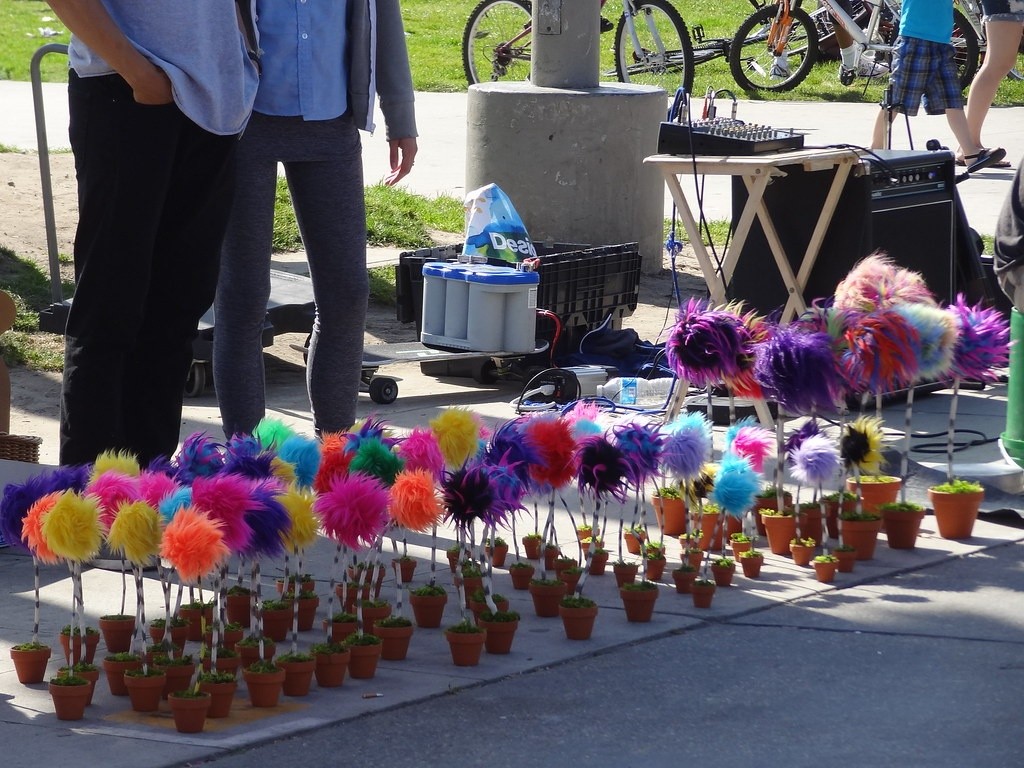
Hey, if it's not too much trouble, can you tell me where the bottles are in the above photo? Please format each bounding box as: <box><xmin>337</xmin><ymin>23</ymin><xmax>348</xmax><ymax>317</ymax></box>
<box><xmin>597</xmin><ymin>377</ymin><xmax>678</xmax><ymax>405</ymax></box>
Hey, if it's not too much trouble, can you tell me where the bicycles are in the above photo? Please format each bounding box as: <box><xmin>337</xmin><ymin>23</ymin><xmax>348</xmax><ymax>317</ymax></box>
<box><xmin>460</xmin><ymin>0</ymin><xmax>695</xmax><ymax>118</ymax></box>
<box><xmin>607</xmin><ymin>0</ymin><xmax>1024</xmax><ymax>104</ymax></box>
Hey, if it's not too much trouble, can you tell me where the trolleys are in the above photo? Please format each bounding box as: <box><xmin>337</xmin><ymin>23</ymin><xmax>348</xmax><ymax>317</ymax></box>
<box><xmin>31</xmin><ymin>43</ymin><xmax>316</xmax><ymax>398</ymax></box>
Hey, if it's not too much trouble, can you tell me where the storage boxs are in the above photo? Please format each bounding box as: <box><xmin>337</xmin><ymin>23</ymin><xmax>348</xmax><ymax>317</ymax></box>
<box><xmin>396</xmin><ymin>239</ymin><xmax>641</xmax><ymax>356</ymax></box>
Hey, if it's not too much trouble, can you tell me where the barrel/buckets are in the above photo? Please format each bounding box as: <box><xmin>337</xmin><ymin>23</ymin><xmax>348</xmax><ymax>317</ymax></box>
<box><xmin>999</xmin><ymin>306</ymin><xmax>1024</xmax><ymax>470</ymax></box>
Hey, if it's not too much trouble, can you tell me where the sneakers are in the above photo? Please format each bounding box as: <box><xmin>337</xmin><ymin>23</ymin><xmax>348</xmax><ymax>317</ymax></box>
<box><xmin>770</xmin><ymin>64</ymin><xmax>792</xmax><ymax>80</ymax></box>
<box><xmin>838</xmin><ymin>57</ymin><xmax>889</xmax><ymax>81</ymax></box>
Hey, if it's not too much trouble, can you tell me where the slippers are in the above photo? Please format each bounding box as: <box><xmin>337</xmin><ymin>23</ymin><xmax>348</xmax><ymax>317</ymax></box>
<box><xmin>956</xmin><ymin>155</ymin><xmax>1011</xmax><ymax>167</ymax></box>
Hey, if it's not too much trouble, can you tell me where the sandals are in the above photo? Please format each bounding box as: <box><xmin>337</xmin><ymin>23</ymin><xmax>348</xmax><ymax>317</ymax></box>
<box><xmin>964</xmin><ymin>147</ymin><xmax>1007</xmax><ymax>173</ymax></box>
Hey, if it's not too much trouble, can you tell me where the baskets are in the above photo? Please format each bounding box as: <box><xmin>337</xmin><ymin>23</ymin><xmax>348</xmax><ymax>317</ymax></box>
<box><xmin>0</xmin><ymin>432</ymin><xmax>43</xmax><ymax>464</ymax></box>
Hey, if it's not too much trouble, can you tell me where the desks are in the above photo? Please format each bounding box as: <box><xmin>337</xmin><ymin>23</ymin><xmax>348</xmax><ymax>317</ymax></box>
<box><xmin>642</xmin><ymin>148</ymin><xmax>872</xmax><ymax>414</ymax></box>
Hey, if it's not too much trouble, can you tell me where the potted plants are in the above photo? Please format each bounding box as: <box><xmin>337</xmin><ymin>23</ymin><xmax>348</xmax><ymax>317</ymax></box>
<box><xmin>9</xmin><ymin>257</ymin><xmax>1012</xmax><ymax>736</ymax></box>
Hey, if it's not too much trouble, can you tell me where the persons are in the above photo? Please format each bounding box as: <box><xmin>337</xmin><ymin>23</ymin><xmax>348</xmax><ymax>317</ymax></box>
<box><xmin>955</xmin><ymin>0</ymin><xmax>1024</xmax><ymax>169</ymax></box>
<box><xmin>46</xmin><ymin>1</ymin><xmax>264</xmax><ymax>466</ymax></box>
<box><xmin>213</xmin><ymin>0</ymin><xmax>418</xmax><ymax>439</ymax></box>
<box><xmin>869</xmin><ymin>0</ymin><xmax>1007</xmax><ymax>174</ymax></box>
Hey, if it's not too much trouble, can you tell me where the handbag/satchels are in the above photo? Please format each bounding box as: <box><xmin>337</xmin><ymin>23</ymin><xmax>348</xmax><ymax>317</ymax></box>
<box><xmin>463</xmin><ymin>183</ymin><xmax>537</xmax><ymax>263</ymax></box>
